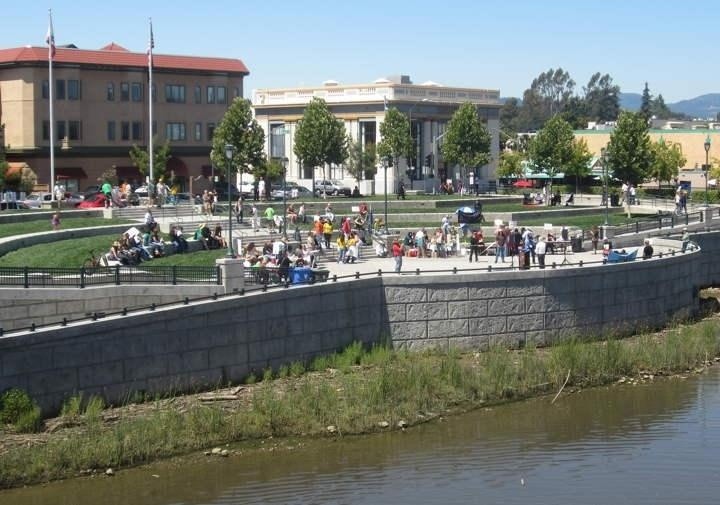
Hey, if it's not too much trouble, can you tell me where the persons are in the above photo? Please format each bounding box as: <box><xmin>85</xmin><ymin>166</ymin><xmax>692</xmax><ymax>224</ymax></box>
<box><xmin>54</xmin><ymin>180</ymin><xmax>66</xmax><ymax>200</ymax></box>
<box><xmin>537</xmin><ymin>185</ymin><xmax>562</xmax><ymax>207</ymax></box>
<box><xmin>50</xmin><ymin>210</ymin><xmax>62</xmax><ymax>231</ymax></box>
<box><xmin>681</xmin><ymin>227</ymin><xmax>690</xmax><ymax>253</ymax></box>
<box><xmin>234</xmin><ymin>176</ymin><xmax>388</xmax><ymax>283</ymax></box>
<box><xmin>467</xmin><ymin>223</ymin><xmax>556</xmax><ymax>269</ymax></box>
<box><xmin>390</xmin><ymin>215</ymin><xmax>457</xmax><ymax>272</ymax></box>
<box><xmin>642</xmin><ymin>239</ymin><xmax>654</xmax><ymax>260</ymax></box>
<box><xmin>620</xmin><ymin>179</ymin><xmax>688</xmax><ymax>216</ymax></box>
<box><xmin>588</xmin><ymin>225</ymin><xmax>601</xmax><ymax>254</ymax></box>
<box><xmin>396</xmin><ymin>176</ymin><xmax>465</xmax><ymax>198</ymax></box>
<box><xmin>100</xmin><ymin>174</ymin><xmax>227</xmax><ymax>265</ymax></box>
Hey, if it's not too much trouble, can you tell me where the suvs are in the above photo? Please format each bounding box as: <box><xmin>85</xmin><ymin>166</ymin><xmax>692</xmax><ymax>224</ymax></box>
<box><xmin>315</xmin><ymin>179</ymin><xmax>352</xmax><ymax>195</ymax></box>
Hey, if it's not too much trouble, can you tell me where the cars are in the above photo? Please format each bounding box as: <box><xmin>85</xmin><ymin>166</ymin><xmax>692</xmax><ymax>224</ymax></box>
<box><xmin>236</xmin><ymin>178</ymin><xmax>321</xmax><ymax>199</ymax></box>
<box><xmin>0</xmin><ymin>183</ymin><xmax>205</xmax><ymax>209</ymax></box>
<box><xmin>512</xmin><ymin>180</ymin><xmax>533</xmax><ymax>187</ymax></box>
<box><xmin>207</xmin><ymin>180</ymin><xmax>250</xmax><ymax>200</ymax></box>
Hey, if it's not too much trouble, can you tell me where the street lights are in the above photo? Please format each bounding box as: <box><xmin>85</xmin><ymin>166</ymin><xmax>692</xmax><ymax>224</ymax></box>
<box><xmin>381</xmin><ymin>153</ymin><xmax>395</xmax><ymax>236</ymax></box>
<box><xmin>602</xmin><ymin>149</ymin><xmax>611</xmax><ymax>225</ymax></box>
<box><xmin>223</xmin><ymin>140</ymin><xmax>237</xmax><ymax>258</ymax></box>
<box><xmin>409</xmin><ymin>96</ymin><xmax>430</xmax><ymax>190</ymax></box>
<box><xmin>703</xmin><ymin>140</ymin><xmax>710</xmax><ymax>206</ymax></box>
<box><xmin>280</xmin><ymin>153</ymin><xmax>291</xmax><ymax>242</ymax></box>
<box><xmin>406</xmin><ymin>164</ymin><xmax>416</xmax><ymax>189</ymax></box>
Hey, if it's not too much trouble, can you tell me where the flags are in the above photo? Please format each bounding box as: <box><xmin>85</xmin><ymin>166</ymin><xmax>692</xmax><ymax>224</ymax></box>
<box><xmin>46</xmin><ymin>16</ymin><xmax>56</xmax><ymax>58</ymax></box>
<box><xmin>145</xmin><ymin>27</ymin><xmax>154</xmax><ymax>64</ymax></box>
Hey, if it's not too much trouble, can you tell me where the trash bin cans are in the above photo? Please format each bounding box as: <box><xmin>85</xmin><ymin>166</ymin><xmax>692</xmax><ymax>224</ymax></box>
<box><xmin>288</xmin><ymin>266</ymin><xmax>330</xmax><ymax>287</ymax></box>
<box><xmin>571</xmin><ymin>235</ymin><xmax>582</xmax><ymax>252</ymax></box>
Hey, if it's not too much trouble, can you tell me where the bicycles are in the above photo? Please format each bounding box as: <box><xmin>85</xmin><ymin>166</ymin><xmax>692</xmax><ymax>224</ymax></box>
<box><xmin>83</xmin><ymin>246</ymin><xmax>114</xmax><ymax>275</ymax></box>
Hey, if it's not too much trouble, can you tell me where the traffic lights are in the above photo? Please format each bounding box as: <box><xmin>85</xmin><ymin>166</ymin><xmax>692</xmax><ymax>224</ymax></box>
<box><xmin>424</xmin><ymin>152</ymin><xmax>431</xmax><ymax>167</ymax></box>
